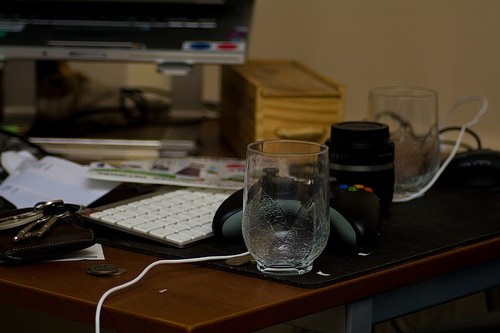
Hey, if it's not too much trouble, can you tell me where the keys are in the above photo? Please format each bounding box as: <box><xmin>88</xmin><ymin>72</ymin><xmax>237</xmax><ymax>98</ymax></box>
<box><xmin>0</xmin><ymin>200</ymin><xmax>80</xmax><ymax>241</ymax></box>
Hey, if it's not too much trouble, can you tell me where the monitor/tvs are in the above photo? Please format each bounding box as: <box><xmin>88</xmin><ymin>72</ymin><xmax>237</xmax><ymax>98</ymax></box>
<box><xmin>0</xmin><ymin>0</ymin><xmax>253</xmax><ymax>159</ymax></box>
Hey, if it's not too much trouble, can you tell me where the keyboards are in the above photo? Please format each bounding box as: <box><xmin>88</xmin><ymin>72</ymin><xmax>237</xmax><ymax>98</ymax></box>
<box><xmin>77</xmin><ymin>183</ymin><xmax>239</xmax><ymax>248</ymax></box>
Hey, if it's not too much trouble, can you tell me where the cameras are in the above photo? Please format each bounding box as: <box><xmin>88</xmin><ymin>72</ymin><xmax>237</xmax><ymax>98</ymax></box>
<box><xmin>318</xmin><ymin>121</ymin><xmax>395</xmax><ymax>239</ymax></box>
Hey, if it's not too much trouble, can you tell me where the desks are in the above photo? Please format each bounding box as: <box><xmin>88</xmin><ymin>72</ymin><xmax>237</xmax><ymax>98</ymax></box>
<box><xmin>0</xmin><ymin>104</ymin><xmax>499</xmax><ymax>333</ymax></box>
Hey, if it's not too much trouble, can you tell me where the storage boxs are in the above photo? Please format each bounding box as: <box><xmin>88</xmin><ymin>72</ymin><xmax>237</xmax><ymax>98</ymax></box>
<box><xmin>219</xmin><ymin>60</ymin><xmax>344</xmax><ymax>165</ymax></box>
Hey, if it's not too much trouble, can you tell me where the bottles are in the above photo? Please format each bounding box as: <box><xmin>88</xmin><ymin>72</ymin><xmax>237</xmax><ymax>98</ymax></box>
<box><xmin>324</xmin><ymin>120</ymin><xmax>394</xmax><ymax>228</ymax></box>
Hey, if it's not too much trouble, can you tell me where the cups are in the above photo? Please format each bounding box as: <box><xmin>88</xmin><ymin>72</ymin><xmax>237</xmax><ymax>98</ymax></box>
<box><xmin>241</xmin><ymin>139</ymin><xmax>331</xmax><ymax>273</ymax></box>
<box><xmin>362</xmin><ymin>86</ymin><xmax>440</xmax><ymax>195</ymax></box>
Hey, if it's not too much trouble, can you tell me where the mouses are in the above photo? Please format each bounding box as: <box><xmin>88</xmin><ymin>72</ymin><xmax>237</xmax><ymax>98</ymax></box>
<box><xmin>439</xmin><ymin>149</ymin><xmax>500</xmax><ymax>193</ymax></box>
